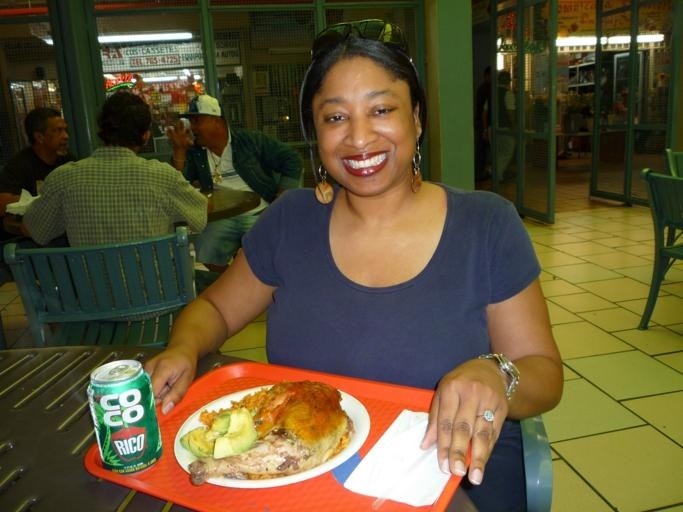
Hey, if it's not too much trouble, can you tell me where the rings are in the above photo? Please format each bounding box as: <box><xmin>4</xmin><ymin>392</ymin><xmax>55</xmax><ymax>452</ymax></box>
<box><xmin>476</xmin><ymin>410</ymin><xmax>494</xmax><ymax>422</ymax></box>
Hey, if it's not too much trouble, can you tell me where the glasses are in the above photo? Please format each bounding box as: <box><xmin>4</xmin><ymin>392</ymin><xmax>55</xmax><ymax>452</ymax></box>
<box><xmin>314</xmin><ymin>19</ymin><xmax>410</xmax><ymax>60</ymax></box>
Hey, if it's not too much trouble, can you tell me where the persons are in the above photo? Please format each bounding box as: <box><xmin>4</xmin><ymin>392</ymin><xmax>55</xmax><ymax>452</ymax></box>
<box><xmin>0</xmin><ymin>108</ymin><xmax>77</xmax><ymax>275</ymax></box>
<box><xmin>143</xmin><ymin>32</ymin><xmax>565</xmax><ymax>509</ymax></box>
<box><xmin>22</xmin><ymin>94</ymin><xmax>212</xmax><ymax>304</ymax></box>
<box><xmin>167</xmin><ymin>92</ymin><xmax>304</xmax><ymax>274</ymax></box>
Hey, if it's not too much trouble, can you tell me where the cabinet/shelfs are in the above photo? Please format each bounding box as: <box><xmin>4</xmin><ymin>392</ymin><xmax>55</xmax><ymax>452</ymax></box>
<box><xmin>569</xmin><ymin>62</ymin><xmax>594</xmax><ymax>96</ymax></box>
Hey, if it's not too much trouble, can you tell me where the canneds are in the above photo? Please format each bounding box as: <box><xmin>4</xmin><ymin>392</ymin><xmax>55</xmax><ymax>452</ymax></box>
<box><xmin>86</xmin><ymin>359</ymin><xmax>163</xmax><ymax>474</ymax></box>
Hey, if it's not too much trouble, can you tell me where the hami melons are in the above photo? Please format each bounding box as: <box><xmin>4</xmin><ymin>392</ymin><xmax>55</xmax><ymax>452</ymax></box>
<box><xmin>179</xmin><ymin>407</ymin><xmax>257</xmax><ymax>459</ymax></box>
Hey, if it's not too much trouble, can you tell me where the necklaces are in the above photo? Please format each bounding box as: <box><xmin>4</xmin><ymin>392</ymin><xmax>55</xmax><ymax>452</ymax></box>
<box><xmin>208</xmin><ymin>146</ymin><xmax>225</xmax><ymax>184</ymax></box>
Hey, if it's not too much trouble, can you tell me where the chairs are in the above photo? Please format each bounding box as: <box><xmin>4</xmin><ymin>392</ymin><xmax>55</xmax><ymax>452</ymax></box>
<box><xmin>664</xmin><ymin>148</ymin><xmax>683</xmax><ymax>177</ymax></box>
<box><xmin>3</xmin><ymin>224</ymin><xmax>199</xmax><ymax>349</ymax></box>
<box><xmin>634</xmin><ymin>167</ymin><xmax>683</xmax><ymax>332</ymax></box>
<box><xmin>272</xmin><ymin>166</ymin><xmax>307</xmax><ymax>191</ymax></box>
<box><xmin>518</xmin><ymin>413</ymin><xmax>555</xmax><ymax>511</ymax></box>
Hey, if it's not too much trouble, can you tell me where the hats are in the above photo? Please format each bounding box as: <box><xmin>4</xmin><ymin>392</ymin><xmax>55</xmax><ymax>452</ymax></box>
<box><xmin>178</xmin><ymin>95</ymin><xmax>224</xmax><ymax>116</ymax></box>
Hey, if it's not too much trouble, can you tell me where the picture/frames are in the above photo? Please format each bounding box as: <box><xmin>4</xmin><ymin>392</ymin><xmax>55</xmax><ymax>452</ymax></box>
<box><xmin>253</xmin><ymin>70</ymin><xmax>269</xmax><ymax>89</ymax></box>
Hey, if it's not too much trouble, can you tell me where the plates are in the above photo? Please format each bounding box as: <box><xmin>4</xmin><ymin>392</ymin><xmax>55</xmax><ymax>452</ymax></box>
<box><xmin>173</xmin><ymin>384</ymin><xmax>370</xmax><ymax>488</ymax></box>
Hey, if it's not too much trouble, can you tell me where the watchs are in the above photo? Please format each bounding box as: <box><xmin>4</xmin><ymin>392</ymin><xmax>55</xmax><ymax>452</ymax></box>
<box><xmin>478</xmin><ymin>350</ymin><xmax>521</xmax><ymax>405</ymax></box>
